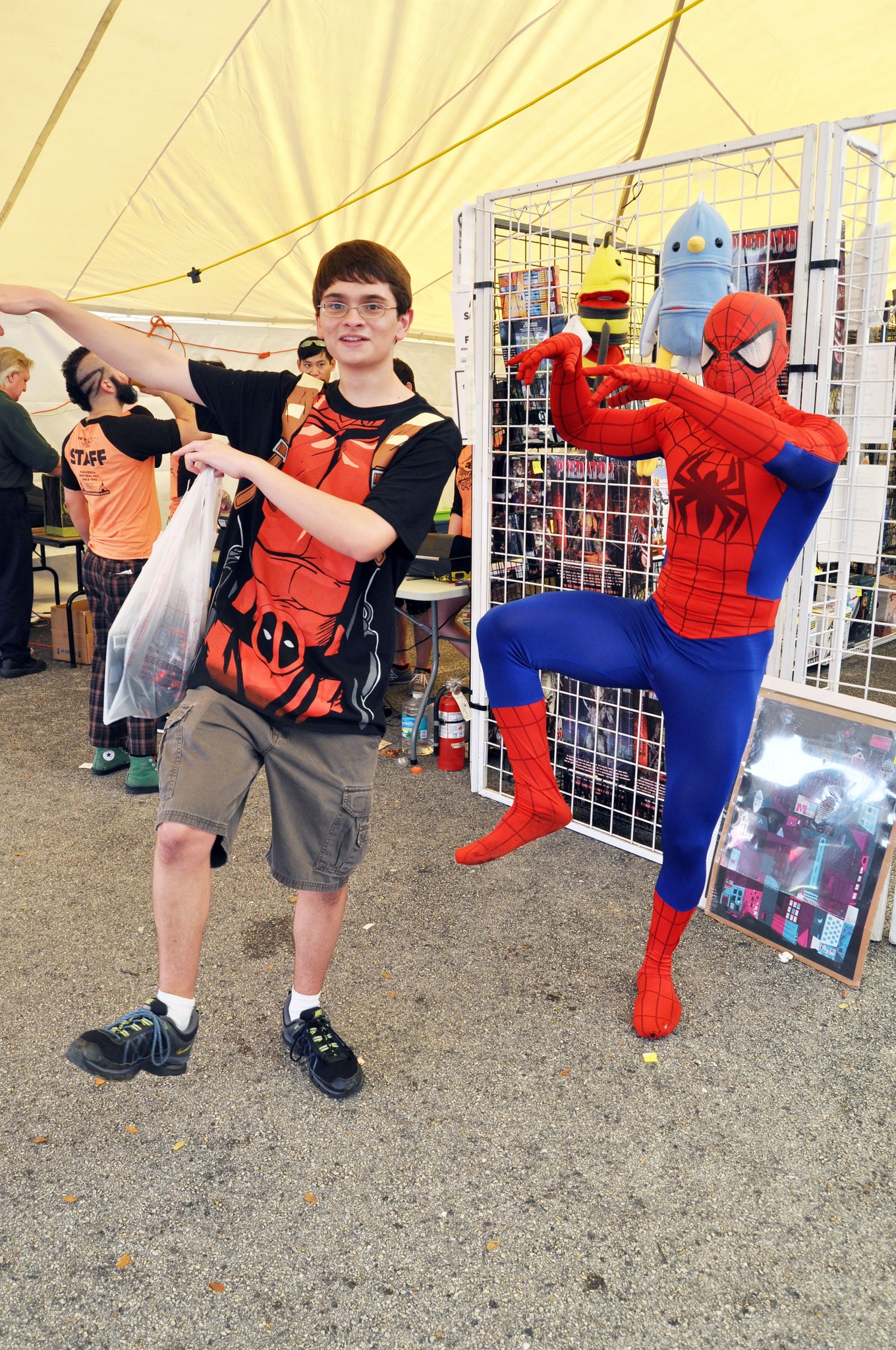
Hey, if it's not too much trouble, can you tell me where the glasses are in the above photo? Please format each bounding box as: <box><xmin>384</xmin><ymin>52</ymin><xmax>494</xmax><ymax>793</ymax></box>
<box><xmin>317</xmin><ymin>302</ymin><xmax>397</xmax><ymax>319</ymax></box>
<box><xmin>299</xmin><ymin>340</ymin><xmax>325</xmax><ymax>350</ymax></box>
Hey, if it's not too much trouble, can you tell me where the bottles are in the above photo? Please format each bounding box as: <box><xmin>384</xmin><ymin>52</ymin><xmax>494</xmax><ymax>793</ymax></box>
<box><xmin>402</xmin><ymin>691</ymin><xmax>434</xmax><ymax>756</ymax></box>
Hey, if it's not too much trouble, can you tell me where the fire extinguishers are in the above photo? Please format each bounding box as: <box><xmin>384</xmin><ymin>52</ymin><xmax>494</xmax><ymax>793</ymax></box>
<box><xmin>432</xmin><ymin>674</ymin><xmax>466</xmax><ymax>771</ymax></box>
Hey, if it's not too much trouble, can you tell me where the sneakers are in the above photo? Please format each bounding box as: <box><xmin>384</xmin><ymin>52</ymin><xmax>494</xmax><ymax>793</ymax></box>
<box><xmin>64</xmin><ymin>997</ymin><xmax>199</xmax><ymax>1081</ymax></box>
<box><xmin>125</xmin><ymin>753</ymin><xmax>159</xmax><ymax>794</ymax></box>
<box><xmin>92</xmin><ymin>744</ymin><xmax>131</xmax><ymax>775</ymax></box>
<box><xmin>0</xmin><ymin>655</ymin><xmax>47</xmax><ymax>678</ymax></box>
<box><xmin>281</xmin><ymin>989</ymin><xmax>365</xmax><ymax>1099</ymax></box>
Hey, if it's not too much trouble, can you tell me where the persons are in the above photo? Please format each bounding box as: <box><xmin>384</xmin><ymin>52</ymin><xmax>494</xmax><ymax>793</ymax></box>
<box><xmin>296</xmin><ymin>336</ymin><xmax>337</xmax><ymax>382</ymax></box>
<box><xmin>428</xmin><ymin>384</ymin><xmax>519</xmax><ymax>666</ymax></box>
<box><xmin>387</xmin><ymin>357</ymin><xmax>440</xmax><ymax>699</ymax></box>
<box><xmin>0</xmin><ymin>239</ymin><xmax>463</xmax><ymax>1100</ymax></box>
<box><xmin>454</xmin><ymin>290</ymin><xmax>853</xmax><ymax>1045</ymax></box>
<box><xmin>61</xmin><ymin>344</ymin><xmax>216</xmax><ymax>795</ymax></box>
<box><xmin>0</xmin><ymin>346</ymin><xmax>63</xmax><ymax>679</ymax></box>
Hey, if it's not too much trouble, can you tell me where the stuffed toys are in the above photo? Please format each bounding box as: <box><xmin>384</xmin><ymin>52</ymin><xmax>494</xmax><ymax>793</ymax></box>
<box><xmin>639</xmin><ymin>190</ymin><xmax>739</xmax><ymax>378</ymax></box>
<box><xmin>559</xmin><ymin>229</ymin><xmax>633</xmax><ymax>397</ymax></box>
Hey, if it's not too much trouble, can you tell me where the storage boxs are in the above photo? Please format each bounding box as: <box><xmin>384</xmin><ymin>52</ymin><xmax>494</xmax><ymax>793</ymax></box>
<box><xmin>49</xmin><ymin>598</ymin><xmax>95</xmax><ymax>665</ymax></box>
<box><xmin>42</xmin><ymin>472</ymin><xmax>80</xmax><ymax>537</ymax></box>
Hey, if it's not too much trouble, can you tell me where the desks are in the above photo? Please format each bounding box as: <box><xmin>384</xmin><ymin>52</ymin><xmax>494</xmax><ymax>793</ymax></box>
<box><xmin>393</xmin><ymin>572</ymin><xmax>471</xmax><ymax>763</ymax></box>
<box><xmin>30</xmin><ymin>526</ymin><xmax>91</xmax><ymax>668</ymax></box>
